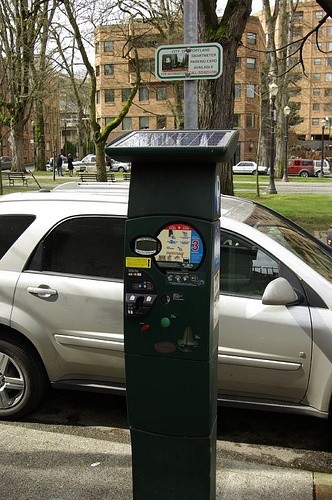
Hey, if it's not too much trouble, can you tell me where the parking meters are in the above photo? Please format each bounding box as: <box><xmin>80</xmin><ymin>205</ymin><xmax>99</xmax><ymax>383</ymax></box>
<box><xmin>104</xmin><ymin>129</ymin><xmax>242</xmax><ymax>499</ymax></box>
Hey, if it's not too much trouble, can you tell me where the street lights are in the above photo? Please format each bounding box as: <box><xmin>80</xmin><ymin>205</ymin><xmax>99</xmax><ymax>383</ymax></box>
<box><xmin>265</xmin><ymin>80</ymin><xmax>278</xmax><ymax>195</ymax></box>
<box><xmin>318</xmin><ymin>120</ymin><xmax>326</xmax><ymax>178</ymax></box>
<box><xmin>281</xmin><ymin>105</ymin><xmax>291</xmax><ymax>182</ymax></box>
<box><xmin>32</xmin><ymin>121</ymin><xmax>37</xmax><ymax>171</ymax></box>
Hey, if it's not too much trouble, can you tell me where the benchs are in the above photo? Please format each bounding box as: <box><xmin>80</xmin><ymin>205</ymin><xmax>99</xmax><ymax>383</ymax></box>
<box><xmin>8</xmin><ymin>172</ymin><xmax>27</xmax><ymax>186</ymax></box>
<box><xmin>122</xmin><ymin>173</ymin><xmax>132</xmax><ymax>181</ymax></box>
<box><xmin>76</xmin><ymin>173</ymin><xmax>115</xmax><ymax>182</ymax></box>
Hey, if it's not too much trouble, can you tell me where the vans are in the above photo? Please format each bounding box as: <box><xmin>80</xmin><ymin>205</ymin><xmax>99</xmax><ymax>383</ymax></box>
<box><xmin>313</xmin><ymin>159</ymin><xmax>330</xmax><ymax>177</ymax></box>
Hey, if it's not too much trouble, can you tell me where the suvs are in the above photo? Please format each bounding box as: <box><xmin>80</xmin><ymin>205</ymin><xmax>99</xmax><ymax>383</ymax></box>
<box><xmin>0</xmin><ymin>182</ymin><xmax>332</xmax><ymax>420</ymax></box>
<box><xmin>266</xmin><ymin>158</ymin><xmax>315</xmax><ymax>177</ymax></box>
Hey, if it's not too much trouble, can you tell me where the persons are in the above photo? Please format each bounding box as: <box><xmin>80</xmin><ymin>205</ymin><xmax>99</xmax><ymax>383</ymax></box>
<box><xmin>56</xmin><ymin>154</ymin><xmax>64</xmax><ymax>176</ymax></box>
<box><xmin>68</xmin><ymin>153</ymin><xmax>74</xmax><ymax>177</ymax></box>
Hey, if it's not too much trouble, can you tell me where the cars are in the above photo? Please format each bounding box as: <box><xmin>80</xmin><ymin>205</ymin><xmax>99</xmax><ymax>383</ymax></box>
<box><xmin>232</xmin><ymin>160</ymin><xmax>269</xmax><ymax>175</ymax></box>
<box><xmin>87</xmin><ymin>156</ymin><xmax>131</xmax><ymax>172</ymax></box>
<box><xmin>0</xmin><ymin>156</ymin><xmax>11</xmax><ymax>171</ymax></box>
